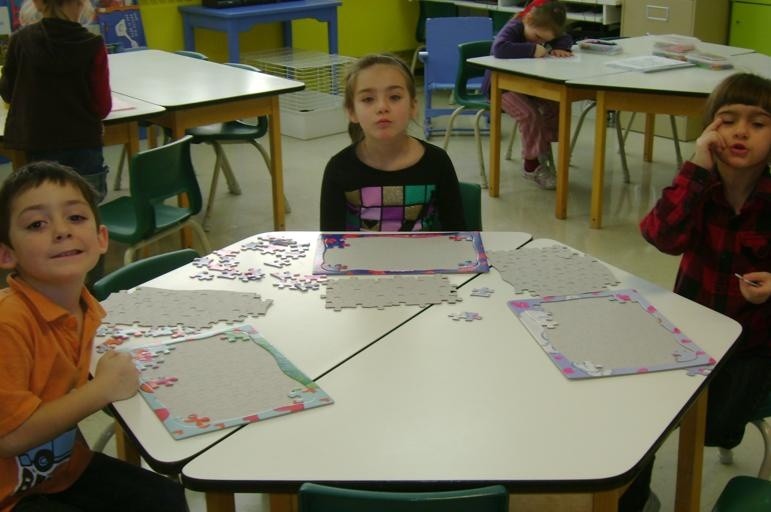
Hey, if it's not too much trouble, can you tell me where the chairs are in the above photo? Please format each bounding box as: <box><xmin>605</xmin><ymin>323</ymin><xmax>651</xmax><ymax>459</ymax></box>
<box><xmin>569</xmin><ymin>37</ymin><xmax>682</xmax><ymax>185</ymax></box>
<box><xmin>419</xmin><ymin>17</ymin><xmax>495</xmax><ymax>140</ymax></box>
<box><xmin>92</xmin><ymin>250</ymin><xmax>199</xmax><ymax>299</ymax></box>
<box><xmin>297</xmin><ymin>483</ymin><xmax>509</xmax><ymax>511</ymax></box>
<box><xmin>713</xmin><ymin>475</ymin><xmax>770</xmax><ymax>511</ymax></box>
<box><xmin>444</xmin><ymin>40</ymin><xmax>556</xmax><ymax>189</ymax></box>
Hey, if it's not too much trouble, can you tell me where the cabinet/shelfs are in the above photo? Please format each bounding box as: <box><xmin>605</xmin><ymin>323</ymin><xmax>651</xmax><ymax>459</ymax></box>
<box><xmin>618</xmin><ymin>0</ymin><xmax>731</xmax><ymax>142</ymax></box>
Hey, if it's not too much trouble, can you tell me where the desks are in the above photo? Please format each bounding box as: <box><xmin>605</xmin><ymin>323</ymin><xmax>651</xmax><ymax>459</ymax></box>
<box><xmin>182</xmin><ymin>239</ymin><xmax>743</xmax><ymax>512</ymax></box>
<box><xmin>565</xmin><ymin>53</ymin><xmax>770</xmax><ymax>228</ymax></box>
<box><xmin>90</xmin><ymin>232</ymin><xmax>532</xmax><ymax>511</ymax></box>
<box><xmin>178</xmin><ymin>0</ymin><xmax>343</xmax><ymax>95</ymax></box>
<box><xmin>465</xmin><ymin>35</ymin><xmax>755</xmax><ymax>219</ymax></box>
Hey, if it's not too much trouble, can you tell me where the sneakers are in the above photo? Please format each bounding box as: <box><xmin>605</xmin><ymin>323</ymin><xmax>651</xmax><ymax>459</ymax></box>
<box><xmin>642</xmin><ymin>491</ymin><xmax>661</xmax><ymax>512</ymax></box>
<box><xmin>520</xmin><ymin>160</ymin><xmax>556</xmax><ymax>189</ymax></box>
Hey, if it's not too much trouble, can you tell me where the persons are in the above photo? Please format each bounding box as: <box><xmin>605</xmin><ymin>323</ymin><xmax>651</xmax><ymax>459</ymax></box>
<box><xmin>481</xmin><ymin>0</ymin><xmax>573</xmax><ymax>190</ymax></box>
<box><xmin>320</xmin><ymin>54</ymin><xmax>465</xmax><ymax>232</ymax></box>
<box><xmin>620</xmin><ymin>73</ymin><xmax>771</xmax><ymax>511</ymax></box>
<box><xmin>0</xmin><ymin>0</ymin><xmax>113</xmax><ymax>207</ymax></box>
<box><xmin>0</xmin><ymin>162</ymin><xmax>187</xmax><ymax>512</ymax></box>
